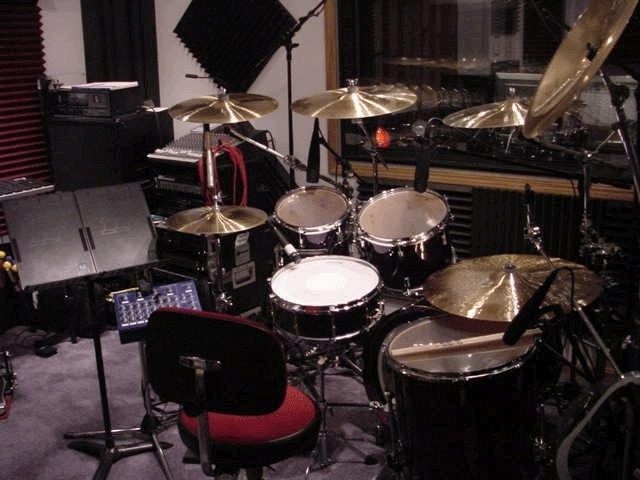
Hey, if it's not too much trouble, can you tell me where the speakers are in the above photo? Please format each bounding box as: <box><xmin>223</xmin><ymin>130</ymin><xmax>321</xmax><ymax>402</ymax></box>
<box><xmin>46</xmin><ymin>105</ymin><xmax>174</xmax><ymax>186</ymax></box>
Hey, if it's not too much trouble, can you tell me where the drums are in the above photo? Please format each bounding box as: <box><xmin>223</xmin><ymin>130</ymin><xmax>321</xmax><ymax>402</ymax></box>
<box><xmin>383</xmin><ymin>314</ymin><xmax>548</xmax><ymax>453</ymax></box>
<box><xmin>353</xmin><ymin>188</ymin><xmax>452</xmax><ymax>297</ymax></box>
<box><xmin>268</xmin><ymin>255</ymin><xmax>385</xmax><ymax>347</ymax></box>
<box><xmin>272</xmin><ymin>185</ymin><xmax>355</xmax><ymax>255</ymax></box>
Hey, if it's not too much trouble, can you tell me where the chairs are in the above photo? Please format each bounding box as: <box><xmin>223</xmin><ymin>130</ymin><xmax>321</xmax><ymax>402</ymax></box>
<box><xmin>141</xmin><ymin>308</ymin><xmax>323</xmax><ymax>480</ymax></box>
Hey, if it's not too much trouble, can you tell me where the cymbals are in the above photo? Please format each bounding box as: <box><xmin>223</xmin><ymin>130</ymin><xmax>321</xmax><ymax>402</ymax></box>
<box><xmin>167</xmin><ymin>93</ymin><xmax>279</xmax><ymax>123</ymax></box>
<box><xmin>394</xmin><ymin>85</ymin><xmax>438</xmax><ymax>113</ymax></box>
<box><xmin>424</xmin><ymin>255</ymin><xmax>608</xmax><ymax>323</ymax></box>
<box><xmin>441</xmin><ymin>96</ymin><xmax>534</xmax><ymax>129</ymax></box>
<box><xmin>166</xmin><ymin>206</ymin><xmax>267</xmax><ymax>234</ymax></box>
<box><xmin>522</xmin><ymin>0</ymin><xmax>637</xmax><ymax>138</ymax></box>
<box><xmin>292</xmin><ymin>83</ymin><xmax>418</xmax><ymax>118</ymax></box>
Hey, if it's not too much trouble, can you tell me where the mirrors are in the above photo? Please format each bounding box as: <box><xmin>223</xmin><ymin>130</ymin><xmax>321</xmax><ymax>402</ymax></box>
<box><xmin>322</xmin><ymin>0</ymin><xmax>640</xmax><ymax>204</ymax></box>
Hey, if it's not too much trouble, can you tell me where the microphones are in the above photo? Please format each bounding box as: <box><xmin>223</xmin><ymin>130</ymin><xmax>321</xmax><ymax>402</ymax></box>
<box><xmin>413</xmin><ymin>136</ymin><xmax>433</xmax><ymax>194</ymax></box>
<box><xmin>185</xmin><ymin>72</ymin><xmax>201</xmax><ymax>80</ymax></box>
<box><xmin>503</xmin><ymin>266</ymin><xmax>560</xmax><ymax>345</ymax></box>
<box><xmin>306</xmin><ymin>129</ymin><xmax>320</xmax><ymax>189</ymax></box>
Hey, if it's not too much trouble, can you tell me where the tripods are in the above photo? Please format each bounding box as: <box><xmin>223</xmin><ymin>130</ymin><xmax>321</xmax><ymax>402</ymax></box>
<box><xmin>62</xmin><ymin>341</ymin><xmax>179</xmax><ymax>479</ymax></box>
<box><xmin>67</xmin><ymin>276</ymin><xmax>175</xmax><ymax>479</ymax></box>
<box><xmin>538</xmin><ymin>276</ymin><xmax>639</xmax><ymax>480</ymax></box>
<box><xmin>278</xmin><ymin>337</ymin><xmax>383</xmax><ymax>480</ymax></box>
